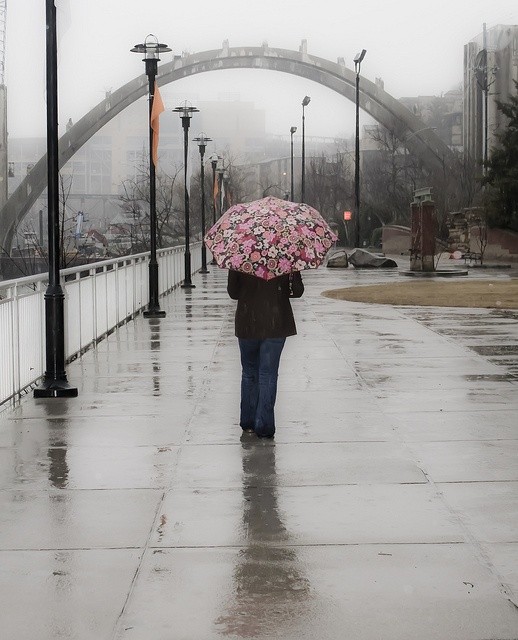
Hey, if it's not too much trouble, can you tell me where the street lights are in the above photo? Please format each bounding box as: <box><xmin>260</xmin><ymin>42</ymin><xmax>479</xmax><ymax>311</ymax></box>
<box><xmin>176</xmin><ymin>98</ymin><xmax>201</xmax><ymax>289</ymax></box>
<box><xmin>217</xmin><ymin>165</ymin><xmax>226</xmax><ymax>246</ymax></box>
<box><xmin>131</xmin><ymin>32</ymin><xmax>173</xmax><ymax>320</ymax></box>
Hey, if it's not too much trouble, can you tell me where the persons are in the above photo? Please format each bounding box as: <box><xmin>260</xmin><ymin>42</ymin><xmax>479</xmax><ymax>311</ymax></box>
<box><xmin>227</xmin><ymin>269</ymin><xmax>305</xmax><ymax>438</ymax></box>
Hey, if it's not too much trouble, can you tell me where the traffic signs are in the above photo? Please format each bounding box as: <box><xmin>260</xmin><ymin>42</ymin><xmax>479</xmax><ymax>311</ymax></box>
<box><xmin>208</xmin><ymin>151</ymin><xmax>221</xmax><ymax>267</ymax></box>
<box><xmin>194</xmin><ymin>136</ymin><xmax>213</xmax><ymax>277</ymax></box>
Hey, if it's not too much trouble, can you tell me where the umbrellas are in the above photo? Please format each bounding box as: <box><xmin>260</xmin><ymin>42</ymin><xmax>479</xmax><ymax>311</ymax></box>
<box><xmin>203</xmin><ymin>194</ymin><xmax>340</xmax><ymax>283</ymax></box>
